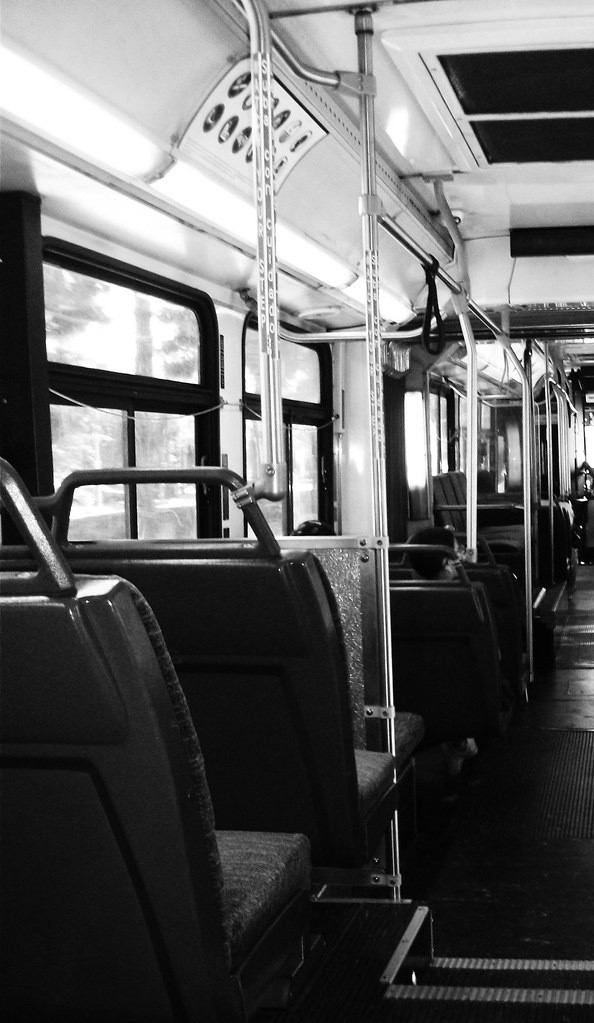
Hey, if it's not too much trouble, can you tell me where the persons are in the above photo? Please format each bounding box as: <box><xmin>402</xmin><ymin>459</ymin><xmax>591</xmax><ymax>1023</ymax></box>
<box><xmin>290</xmin><ymin>520</ymin><xmax>336</xmax><ymax>536</ymax></box>
<box><xmin>407</xmin><ymin>527</ymin><xmax>478</xmax><ymax>775</ymax></box>
<box><xmin>542</xmin><ymin>474</ymin><xmax>582</xmax><ymax>599</ymax></box>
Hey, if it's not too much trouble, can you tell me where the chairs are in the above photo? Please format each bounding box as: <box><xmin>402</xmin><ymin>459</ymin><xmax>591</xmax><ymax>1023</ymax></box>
<box><xmin>0</xmin><ymin>458</ymin><xmax>564</xmax><ymax>1023</ymax></box>
